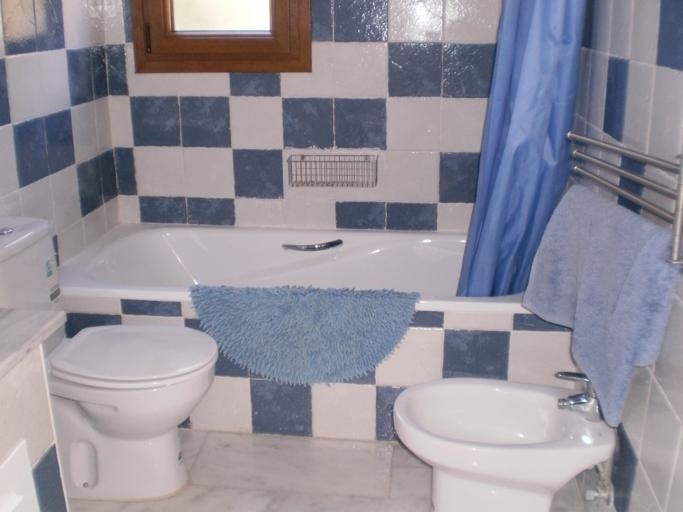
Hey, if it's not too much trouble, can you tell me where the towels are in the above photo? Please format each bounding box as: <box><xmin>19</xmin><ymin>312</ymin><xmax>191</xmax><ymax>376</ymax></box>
<box><xmin>522</xmin><ymin>186</ymin><xmax>676</xmax><ymax>427</ymax></box>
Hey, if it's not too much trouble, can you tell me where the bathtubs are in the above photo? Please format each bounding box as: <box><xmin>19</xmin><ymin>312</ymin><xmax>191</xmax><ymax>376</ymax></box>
<box><xmin>59</xmin><ymin>224</ymin><xmax>530</xmax><ymax>315</ymax></box>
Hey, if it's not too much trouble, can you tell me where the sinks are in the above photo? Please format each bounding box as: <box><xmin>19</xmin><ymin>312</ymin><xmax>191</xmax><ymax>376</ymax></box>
<box><xmin>392</xmin><ymin>375</ymin><xmax>615</xmax><ymax>512</ymax></box>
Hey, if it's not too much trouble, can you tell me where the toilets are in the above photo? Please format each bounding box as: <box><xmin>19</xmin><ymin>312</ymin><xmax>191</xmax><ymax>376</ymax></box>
<box><xmin>0</xmin><ymin>217</ymin><xmax>218</xmax><ymax>501</ymax></box>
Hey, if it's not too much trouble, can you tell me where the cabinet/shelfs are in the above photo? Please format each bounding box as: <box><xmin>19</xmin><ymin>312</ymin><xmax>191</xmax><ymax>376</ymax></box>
<box><xmin>0</xmin><ymin>345</ymin><xmax>71</xmax><ymax>512</ymax></box>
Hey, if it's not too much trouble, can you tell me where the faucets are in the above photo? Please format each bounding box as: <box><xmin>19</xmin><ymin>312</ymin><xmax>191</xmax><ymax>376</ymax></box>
<box><xmin>554</xmin><ymin>371</ymin><xmax>601</xmax><ymax>422</ymax></box>
